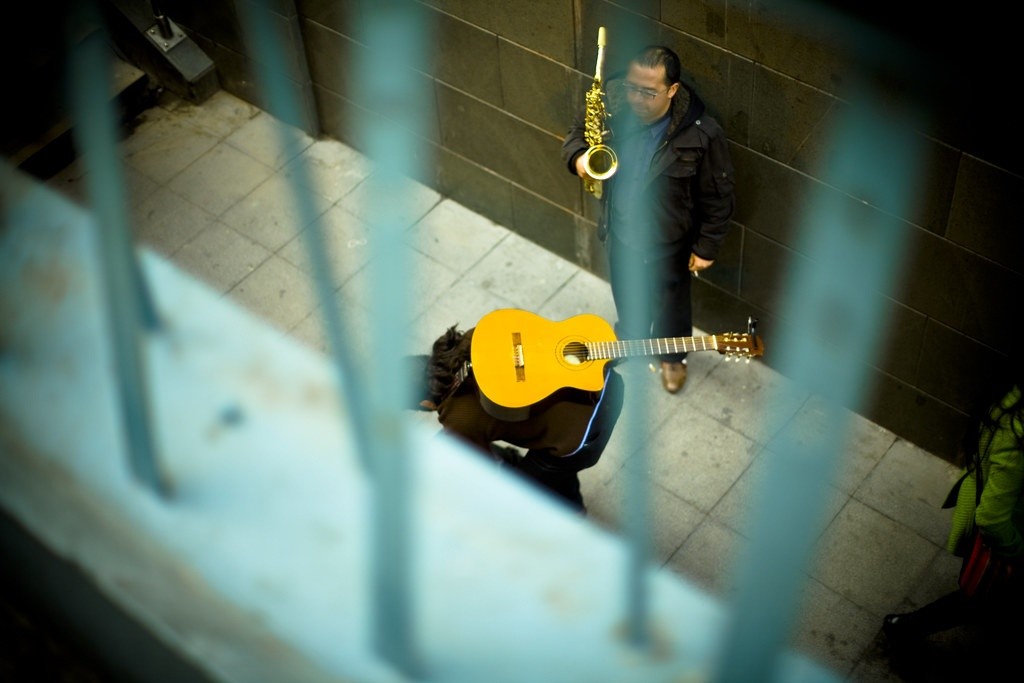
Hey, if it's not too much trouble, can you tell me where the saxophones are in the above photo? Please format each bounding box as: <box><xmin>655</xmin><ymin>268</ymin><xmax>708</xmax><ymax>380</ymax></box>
<box><xmin>577</xmin><ymin>27</ymin><xmax>619</xmax><ymax>200</ymax></box>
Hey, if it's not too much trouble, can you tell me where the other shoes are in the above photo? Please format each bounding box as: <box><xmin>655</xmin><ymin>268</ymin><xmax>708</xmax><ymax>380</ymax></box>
<box><xmin>886</xmin><ymin>614</ymin><xmax>936</xmax><ymax>649</ymax></box>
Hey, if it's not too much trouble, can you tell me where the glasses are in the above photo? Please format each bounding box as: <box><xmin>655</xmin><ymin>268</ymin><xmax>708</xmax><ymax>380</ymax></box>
<box><xmin>622</xmin><ymin>77</ymin><xmax>669</xmax><ymax>99</ymax></box>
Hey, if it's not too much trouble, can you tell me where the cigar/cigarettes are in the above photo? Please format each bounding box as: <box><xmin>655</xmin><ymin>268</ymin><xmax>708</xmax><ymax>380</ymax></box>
<box><xmin>693</xmin><ymin>270</ymin><xmax>698</xmax><ymax>277</ymax></box>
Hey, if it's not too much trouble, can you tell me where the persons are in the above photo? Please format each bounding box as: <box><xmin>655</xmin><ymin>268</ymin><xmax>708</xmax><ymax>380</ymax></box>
<box><xmin>559</xmin><ymin>43</ymin><xmax>735</xmax><ymax>393</ymax></box>
<box><xmin>399</xmin><ymin>322</ymin><xmax>625</xmax><ymax>517</ymax></box>
<box><xmin>941</xmin><ymin>377</ymin><xmax>1024</xmax><ymax>583</ymax></box>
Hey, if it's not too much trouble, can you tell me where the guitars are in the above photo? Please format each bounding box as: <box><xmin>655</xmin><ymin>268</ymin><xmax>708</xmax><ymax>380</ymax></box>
<box><xmin>468</xmin><ymin>309</ymin><xmax>766</xmax><ymax>424</ymax></box>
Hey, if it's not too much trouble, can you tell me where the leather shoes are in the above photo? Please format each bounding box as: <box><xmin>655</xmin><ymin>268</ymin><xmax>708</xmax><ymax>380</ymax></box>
<box><xmin>662</xmin><ymin>360</ymin><xmax>686</xmax><ymax>392</ymax></box>
